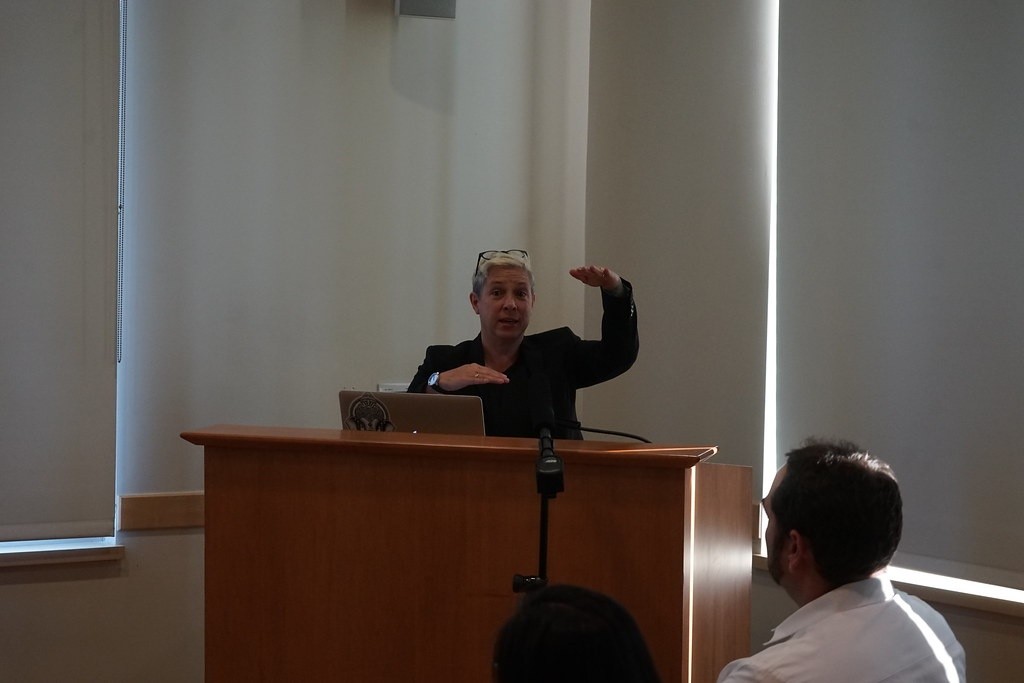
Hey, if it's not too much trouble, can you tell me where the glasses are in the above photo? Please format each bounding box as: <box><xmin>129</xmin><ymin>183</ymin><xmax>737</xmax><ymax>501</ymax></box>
<box><xmin>475</xmin><ymin>249</ymin><xmax>528</xmax><ymax>277</ymax></box>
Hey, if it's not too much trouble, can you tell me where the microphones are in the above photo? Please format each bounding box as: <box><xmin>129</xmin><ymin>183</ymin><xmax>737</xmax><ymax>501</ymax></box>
<box><xmin>531</xmin><ymin>372</ymin><xmax>565</xmax><ymax>498</ymax></box>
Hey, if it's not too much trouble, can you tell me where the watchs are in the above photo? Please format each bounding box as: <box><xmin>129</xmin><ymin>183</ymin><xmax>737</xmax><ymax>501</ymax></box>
<box><xmin>428</xmin><ymin>370</ymin><xmax>448</xmax><ymax>394</ymax></box>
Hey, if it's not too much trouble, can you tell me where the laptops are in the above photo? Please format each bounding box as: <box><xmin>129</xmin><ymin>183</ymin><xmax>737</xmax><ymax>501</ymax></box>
<box><xmin>340</xmin><ymin>390</ymin><xmax>485</xmax><ymax>436</ymax></box>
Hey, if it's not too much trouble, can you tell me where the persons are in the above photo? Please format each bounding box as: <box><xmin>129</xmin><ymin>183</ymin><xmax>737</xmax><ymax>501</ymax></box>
<box><xmin>493</xmin><ymin>584</ymin><xmax>662</xmax><ymax>683</ymax></box>
<box><xmin>406</xmin><ymin>250</ymin><xmax>639</xmax><ymax>442</ymax></box>
<box><xmin>715</xmin><ymin>437</ymin><xmax>966</xmax><ymax>683</ymax></box>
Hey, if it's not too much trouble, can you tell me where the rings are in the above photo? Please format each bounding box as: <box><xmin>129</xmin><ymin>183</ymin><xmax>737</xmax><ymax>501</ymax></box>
<box><xmin>475</xmin><ymin>372</ymin><xmax>480</xmax><ymax>379</ymax></box>
<box><xmin>600</xmin><ymin>267</ymin><xmax>605</xmax><ymax>280</ymax></box>
